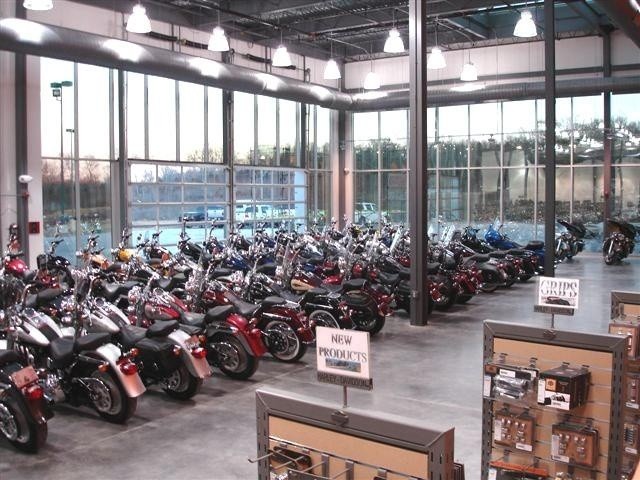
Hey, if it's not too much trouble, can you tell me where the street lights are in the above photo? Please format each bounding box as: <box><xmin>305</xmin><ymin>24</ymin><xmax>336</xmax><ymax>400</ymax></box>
<box><xmin>260</xmin><ymin>154</ymin><xmax>267</xmax><ymax>199</ymax></box>
<box><xmin>49</xmin><ymin>79</ymin><xmax>73</xmax><ymax>226</ymax></box>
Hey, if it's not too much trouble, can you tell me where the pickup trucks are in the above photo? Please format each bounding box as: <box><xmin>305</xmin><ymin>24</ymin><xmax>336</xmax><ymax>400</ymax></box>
<box><xmin>177</xmin><ymin>199</ymin><xmax>391</xmax><ymax>228</ymax></box>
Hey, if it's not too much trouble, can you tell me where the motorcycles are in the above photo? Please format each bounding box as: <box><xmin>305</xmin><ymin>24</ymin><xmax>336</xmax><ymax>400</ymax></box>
<box><xmin>554</xmin><ymin>218</ymin><xmax>597</xmax><ymax>263</ymax></box>
<box><xmin>602</xmin><ymin>217</ymin><xmax>636</xmax><ymax>264</ymax></box>
